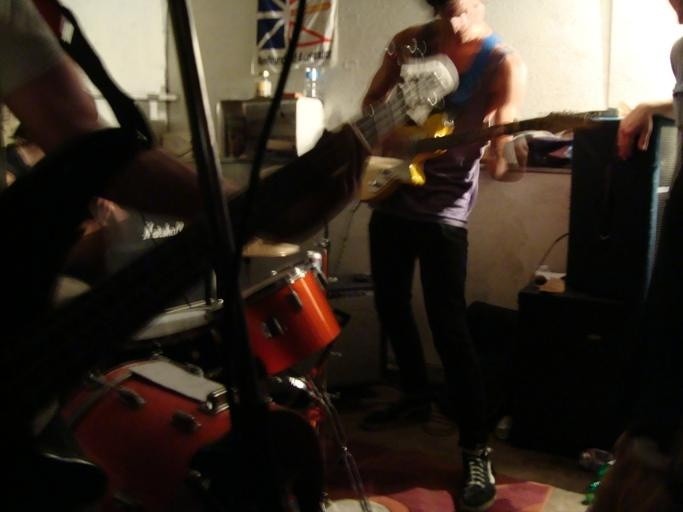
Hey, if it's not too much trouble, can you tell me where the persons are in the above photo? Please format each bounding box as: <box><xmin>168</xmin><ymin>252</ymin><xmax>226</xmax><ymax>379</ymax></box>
<box><xmin>569</xmin><ymin>1</ymin><xmax>682</xmax><ymax>478</ymax></box>
<box><xmin>359</xmin><ymin>1</ymin><xmax>531</xmax><ymax>509</ymax></box>
<box><xmin>1</xmin><ymin>2</ymin><xmax>366</xmax><ymax>452</ymax></box>
<box><xmin>585</xmin><ymin>424</ymin><xmax>683</xmax><ymax>512</ymax></box>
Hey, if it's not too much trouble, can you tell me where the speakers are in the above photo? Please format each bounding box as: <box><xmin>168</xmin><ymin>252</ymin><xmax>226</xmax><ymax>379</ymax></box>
<box><xmin>567</xmin><ymin>116</ymin><xmax>662</xmax><ymax>301</ymax></box>
<box><xmin>519</xmin><ymin>271</ymin><xmax>642</xmax><ymax>461</ymax></box>
<box><xmin>437</xmin><ymin>301</ymin><xmax>519</xmax><ymax>428</ymax></box>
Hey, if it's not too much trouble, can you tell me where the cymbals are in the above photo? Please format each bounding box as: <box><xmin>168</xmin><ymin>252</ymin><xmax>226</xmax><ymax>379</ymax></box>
<box><xmin>241</xmin><ymin>241</ymin><xmax>298</xmax><ymax>257</ymax></box>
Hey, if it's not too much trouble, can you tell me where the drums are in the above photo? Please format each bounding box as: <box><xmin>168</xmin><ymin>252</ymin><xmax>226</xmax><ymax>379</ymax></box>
<box><xmin>262</xmin><ymin>360</ymin><xmax>326</xmax><ymax>429</ymax></box>
<box><xmin>123</xmin><ymin>299</ymin><xmax>241</xmax><ymax>406</ymax></box>
<box><xmin>241</xmin><ymin>261</ymin><xmax>343</xmax><ymax>374</ymax></box>
<box><xmin>54</xmin><ymin>352</ymin><xmax>332</xmax><ymax>511</ymax></box>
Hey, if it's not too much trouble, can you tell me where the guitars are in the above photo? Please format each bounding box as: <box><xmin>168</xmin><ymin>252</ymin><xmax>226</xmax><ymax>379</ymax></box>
<box><xmin>358</xmin><ymin>111</ymin><xmax>616</xmax><ymax>200</ymax></box>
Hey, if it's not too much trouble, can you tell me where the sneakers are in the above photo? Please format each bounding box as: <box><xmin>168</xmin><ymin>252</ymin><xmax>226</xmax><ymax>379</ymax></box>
<box><xmin>459</xmin><ymin>446</ymin><xmax>497</xmax><ymax>512</ymax></box>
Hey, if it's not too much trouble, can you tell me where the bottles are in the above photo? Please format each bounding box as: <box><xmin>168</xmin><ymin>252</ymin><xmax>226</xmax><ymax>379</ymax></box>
<box><xmin>302</xmin><ymin>54</ymin><xmax>318</xmax><ymax>100</ymax></box>
<box><xmin>256</xmin><ymin>71</ymin><xmax>273</xmax><ymax>100</ymax></box>
